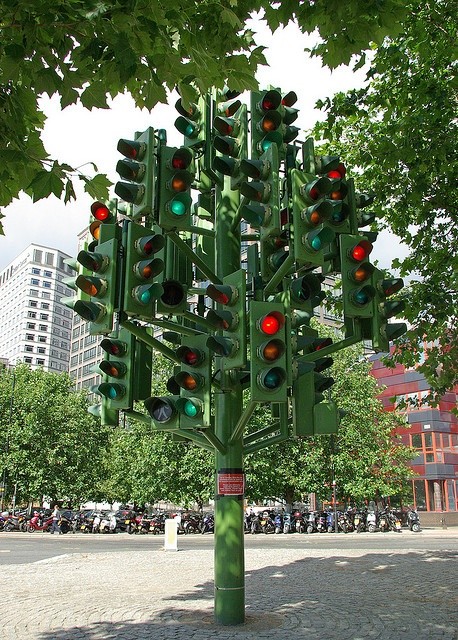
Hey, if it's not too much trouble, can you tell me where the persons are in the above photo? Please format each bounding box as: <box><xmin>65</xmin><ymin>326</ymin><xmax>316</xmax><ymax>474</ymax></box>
<box><xmin>50</xmin><ymin>505</ymin><xmax>62</xmax><ymax>534</ymax></box>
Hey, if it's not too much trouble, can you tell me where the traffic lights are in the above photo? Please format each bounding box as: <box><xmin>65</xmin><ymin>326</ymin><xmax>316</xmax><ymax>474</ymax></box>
<box><xmin>88</xmin><ymin>196</ymin><xmax>118</xmax><ymax>240</ymax></box>
<box><xmin>284</xmin><ymin>273</ymin><xmax>322</xmax><ymax>311</ymax></box>
<box><xmin>260</xmin><ymin>227</ymin><xmax>289</xmax><ymax>282</ymax></box>
<box><xmin>143</xmin><ymin>395</ymin><xmax>180</xmax><ymax>431</ymax></box>
<box><xmin>174</xmin><ymin>81</ymin><xmax>207</xmax><ymax>148</ymax></box>
<box><xmin>339</xmin><ymin>232</ymin><xmax>374</xmax><ymax>319</ymax></box>
<box><xmin>98</xmin><ymin>330</ymin><xmax>133</xmax><ymax>410</ymax></box>
<box><xmin>301</xmin><ymin>325</ymin><xmax>334</xmax><ymax>393</ymax></box>
<box><xmin>123</xmin><ymin>220</ymin><xmax>167</xmax><ymax>319</ymax></box>
<box><xmin>117</xmin><ymin>201</ymin><xmax>134</xmax><ymax>219</ymax></box>
<box><xmin>248</xmin><ymin>300</ymin><xmax>288</xmax><ymax>403</ymax></box>
<box><xmin>289</xmin><ymin>169</ymin><xmax>334</xmax><ymax>265</ymax></box>
<box><xmin>213</xmin><ymin>102</ymin><xmax>249</xmax><ymax>191</ymax></box>
<box><xmin>239</xmin><ymin>142</ymin><xmax>283</xmax><ymax>238</ymax></box>
<box><xmin>203</xmin><ymin>269</ymin><xmax>246</xmax><ymax>371</ymax></box>
<box><xmin>212</xmin><ymin>83</ymin><xmax>241</xmax><ymax>118</ymax></box>
<box><xmin>60</xmin><ymin>241</ymin><xmax>94</xmax><ymax>312</ymax></box>
<box><xmin>320</xmin><ymin>158</ymin><xmax>351</xmax><ymax>233</ymax></box>
<box><xmin>158</xmin><ymin>145</ymin><xmax>193</xmax><ymax>232</ymax></box>
<box><xmin>87</xmin><ymin>361</ymin><xmax>119</xmax><ymax>427</ymax></box>
<box><xmin>157</xmin><ymin>278</ymin><xmax>189</xmax><ymax>316</ymax></box>
<box><xmin>370</xmin><ymin>264</ymin><xmax>408</xmax><ymax>350</ymax></box>
<box><xmin>267</xmin><ymin>83</ymin><xmax>301</xmax><ymax>162</ymax></box>
<box><xmin>174</xmin><ymin>333</ymin><xmax>211</xmax><ymax>428</ymax></box>
<box><xmin>72</xmin><ymin>238</ymin><xmax>118</xmax><ymax>336</ymax></box>
<box><xmin>250</xmin><ymin>87</ymin><xmax>283</xmax><ymax>161</ymax></box>
<box><xmin>114</xmin><ymin>126</ymin><xmax>156</xmax><ymax>221</ymax></box>
<box><xmin>302</xmin><ymin>137</ymin><xmax>340</xmax><ymax>177</ymax></box>
<box><xmin>347</xmin><ymin>176</ymin><xmax>379</xmax><ymax>242</ymax></box>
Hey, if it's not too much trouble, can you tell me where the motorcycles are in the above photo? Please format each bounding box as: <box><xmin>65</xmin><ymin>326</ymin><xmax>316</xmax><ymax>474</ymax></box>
<box><xmin>243</xmin><ymin>500</ymin><xmax>423</xmax><ymax>534</ymax></box>
<box><xmin>0</xmin><ymin>504</ymin><xmax>215</xmax><ymax>535</ymax></box>
